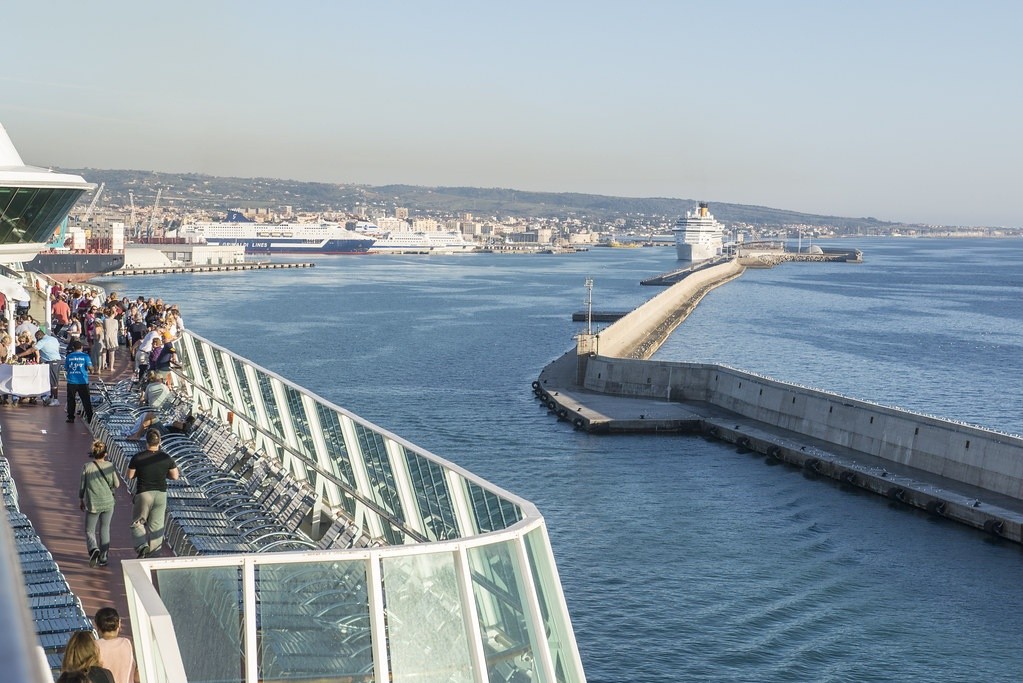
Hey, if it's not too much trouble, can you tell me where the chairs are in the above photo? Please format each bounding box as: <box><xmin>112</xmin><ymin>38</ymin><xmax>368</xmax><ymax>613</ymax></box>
<box><xmin>0</xmin><ymin>379</ymin><xmax>484</xmax><ymax>682</ymax></box>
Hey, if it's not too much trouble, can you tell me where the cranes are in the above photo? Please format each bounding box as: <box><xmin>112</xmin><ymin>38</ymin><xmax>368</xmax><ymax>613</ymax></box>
<box><xmin>80</xmin><ymin>180</ymin><xmax>174</xmax><ymax>244</ymax></box>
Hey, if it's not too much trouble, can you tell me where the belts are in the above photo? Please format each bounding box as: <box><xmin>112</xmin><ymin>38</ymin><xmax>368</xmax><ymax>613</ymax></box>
<box><xmin>139</xmin><ymin>349</ymin><xmax>149</xmax><ymax>355</ymax></box>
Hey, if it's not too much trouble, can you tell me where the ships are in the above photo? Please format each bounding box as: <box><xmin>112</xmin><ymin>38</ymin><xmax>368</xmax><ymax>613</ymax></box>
<box><xmin>671</xmin><ymin>200</ymin><xmax>725</xmax><ymax>261</ymax></box>
<box><xmin>164</xmin><ymin>209</ymin><xmax>478</xmax><ymax>258</ymax></box>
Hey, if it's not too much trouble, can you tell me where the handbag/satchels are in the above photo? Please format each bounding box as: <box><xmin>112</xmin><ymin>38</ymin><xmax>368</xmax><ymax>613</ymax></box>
<box><xmin>92</xmin><ymin>460</ymin><xmax>117</xmax><ymax>503</ymax></box>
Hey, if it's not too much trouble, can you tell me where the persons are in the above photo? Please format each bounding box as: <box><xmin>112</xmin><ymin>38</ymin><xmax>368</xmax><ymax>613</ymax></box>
<box><xmin>1</xmin><ymin>278</ymin><xmax>186</xmax><ymax>683</ymax></box>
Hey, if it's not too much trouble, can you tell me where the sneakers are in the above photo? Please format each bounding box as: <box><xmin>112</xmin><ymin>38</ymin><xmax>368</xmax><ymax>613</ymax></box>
<box><xmin>44</xmin><ymin>398</ymin><xmax>60</xmax><ymax>407</ymax></box>
<box><xmin>136</xmin><ymin>544</ymin><xmax>149</xmax><ymax>558</ymax></box>
<box><xmin>89</xmin><ymin>548</ymin><xmax>100</xmax><ymax>568</ymax></box>
<box><xmin>98</xmin><ymin>555</ymin><xmax>109</xmax><ymax>567</ymax></box>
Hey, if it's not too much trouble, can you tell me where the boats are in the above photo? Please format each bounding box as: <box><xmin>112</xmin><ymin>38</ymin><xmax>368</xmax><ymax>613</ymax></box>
<box><xmin>0</xmin><ymin>120</ymin><xmax>587</xmax><ymax>683</ymax></box>
<box><xmin>22</xmin><ymin>252</ymin><xmax>126</xmax><ymax>282</ymax></box>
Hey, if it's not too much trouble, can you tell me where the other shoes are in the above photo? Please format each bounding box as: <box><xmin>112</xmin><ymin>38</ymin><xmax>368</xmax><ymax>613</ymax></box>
<box><xmin>3</xmin><ymin>401</ymin><xmax>9</xmax><ymax>407</ymax></box>
<box><xmin>29</xmin><ymin>399</ymin><xmax>37</xmax><ymax>405</ymax></box>
<box><xmin>11</xmin><ymin>401</ymin><xmax>23</xmax><ymax>407</ymax></box>
<box><xmin>66</xmin><ymin>419</ymin><xmax>74</xmax><ymax>423</ymax></box>
